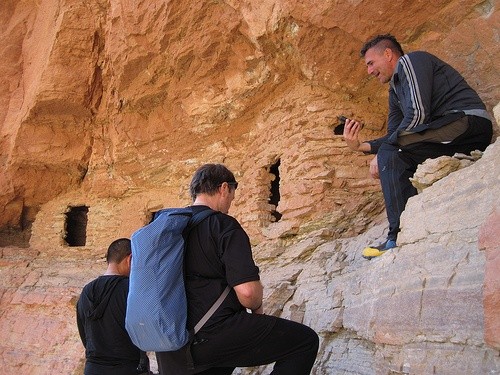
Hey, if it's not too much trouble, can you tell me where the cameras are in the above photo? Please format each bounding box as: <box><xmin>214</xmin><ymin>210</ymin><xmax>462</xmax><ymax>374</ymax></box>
<box><xmin>333</xmin><ymin>116</ymin><xmax>364</xmax><ymax>135</ymax></box>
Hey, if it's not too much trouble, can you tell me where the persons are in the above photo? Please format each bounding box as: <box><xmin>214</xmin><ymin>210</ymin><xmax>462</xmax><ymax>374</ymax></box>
<box><xmin>76</xmin><ymin>238</ymin><xmax>153</xmax><ymax>375</ymax></box>
<box><xmin>155</xmin><ymin>164</ymin><xmax>319</xmax><ymax>375</ymax></box>
<box><xmin>343</xmin><ymin>33</ymin><xmax>493</xmax><ymax>260</ymax></box>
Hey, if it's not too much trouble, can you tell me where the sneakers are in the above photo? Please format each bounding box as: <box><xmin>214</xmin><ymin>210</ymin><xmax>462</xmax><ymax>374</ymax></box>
<box><xmin>362</xmin><ymin>239</ymin><xmax>396</xmax><ymax>259</ymax></box>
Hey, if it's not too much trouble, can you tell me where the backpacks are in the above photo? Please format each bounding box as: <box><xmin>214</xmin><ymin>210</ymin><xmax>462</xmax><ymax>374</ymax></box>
<box><xmin>124</xmin><ymin>208</ymin><xmax>233</xmax><ymax>352</ymax></box>
